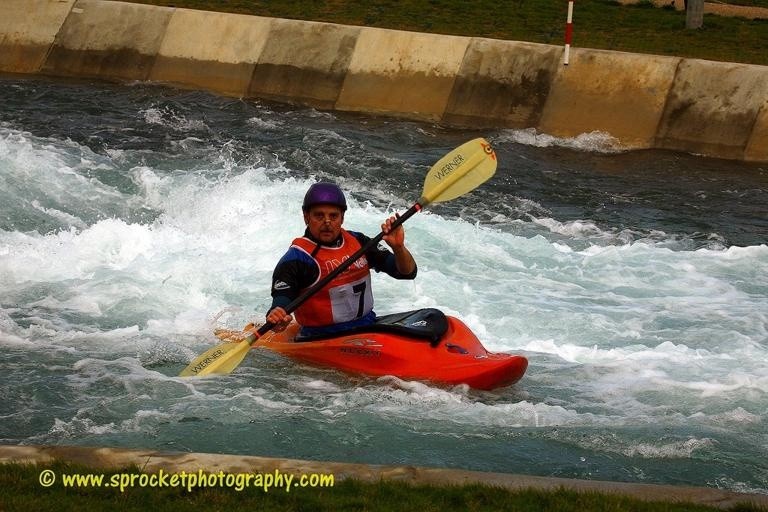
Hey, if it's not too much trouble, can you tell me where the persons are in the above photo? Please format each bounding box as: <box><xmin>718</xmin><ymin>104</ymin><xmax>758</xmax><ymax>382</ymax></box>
<box><xmin>266</xmin><ymin>181</ymin><xmax>417</xmax><ymax>342</ymax></box>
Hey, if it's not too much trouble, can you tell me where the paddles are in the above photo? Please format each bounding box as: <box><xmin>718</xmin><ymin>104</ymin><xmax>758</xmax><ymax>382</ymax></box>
<box><xmin>178</xmin><ymin>138</ymin><xmax>497</xmax><ymax>376</ymax></box>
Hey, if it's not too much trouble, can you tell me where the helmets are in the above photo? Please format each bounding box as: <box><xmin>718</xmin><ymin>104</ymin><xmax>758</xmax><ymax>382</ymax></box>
<box><xmin>302</xmin><ymin>182</ymin><xmax>347</xmax><ymax>211</ymax></box>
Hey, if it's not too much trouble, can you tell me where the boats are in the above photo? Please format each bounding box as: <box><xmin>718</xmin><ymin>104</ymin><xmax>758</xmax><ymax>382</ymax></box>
<box><xmin>215</xmin><ymin>311</ymin><xmax>529</xmax><ymax>391</ymax></box>
